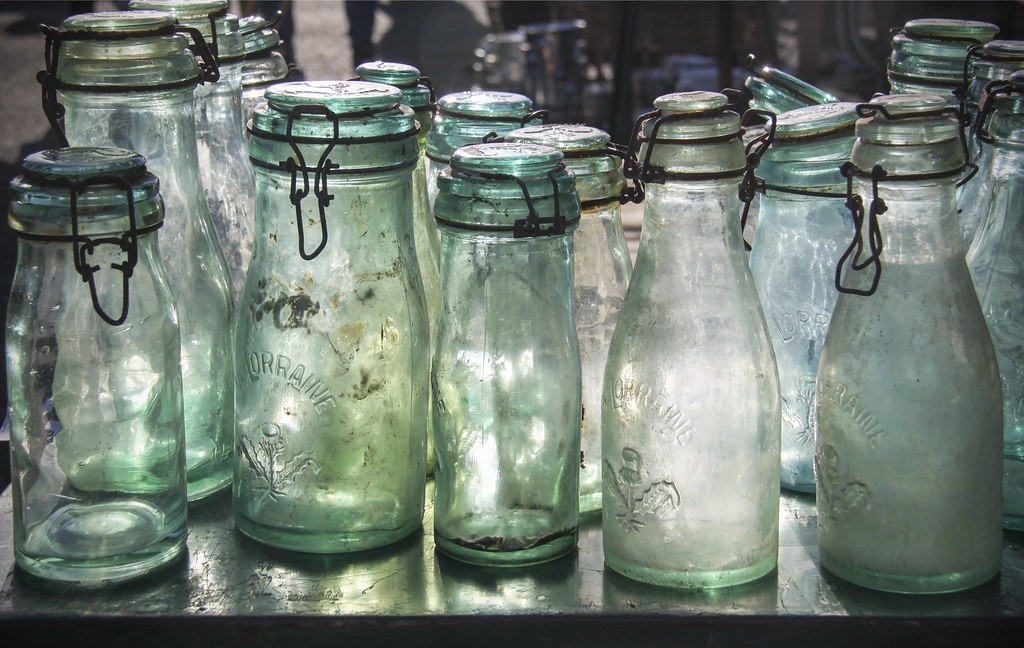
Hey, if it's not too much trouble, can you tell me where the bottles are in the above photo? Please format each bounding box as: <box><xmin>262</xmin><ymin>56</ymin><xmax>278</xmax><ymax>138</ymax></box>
<box><xmin>600</xmin><ymin>90</ymin><xmax>779</xmax><ymax>589</ymax></box>
<box><xmin>886</xmin><ymin>16</ymin><xmax>1000</xmax><ymax>97</ymax></box>
<box><xmin>7</xmin><ymin>146</ymin><xmax>188</xmax><ymax>585</ymax></box>
<box><xmin>348</xmin><ymin>60</ymin><xmax>448</xmax><ymax>485</ymax></box>
<box><xmin>741</xmin><ymin>64</ymin><xmax>864</xmax><ymax>497</ymax></box>
<box><xmin>505</xmin><ymin>123</ymin><xmax>636</xmax><ymax>519</ymax></box>
<box><xmin>434</xmin><ymin>145</ymin><xmax>582</xmax><ymax>569</ymax></box>
<box><xmin>955</xmin><ymin>39</ymin><xmax>1024</xmax><ymax>462</ymax></box>
<box><xmin>426</xmin><ymin>92</ymin><xmax>547</xmax><ymax>207</ymax></box>
<box><xmin>231</xmin><ymin>82</ymin><xmax>432</xmax><ymax>554</ymax></box>
<box><xmin>49</xmin><ymin>0</ymin><xmax>288</xmax><ymax>503</ymax></box>
<box><xmin>813</xmin><ymin>92</ymin><xmax>1004</xmax><ymax>594</ymax></box>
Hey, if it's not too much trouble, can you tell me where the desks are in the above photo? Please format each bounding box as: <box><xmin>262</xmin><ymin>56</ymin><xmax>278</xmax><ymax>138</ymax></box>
<box><xmin>0</xmin><ymin>480</ymin><xmax>1024</xmax><ymax>648</ymax></box>
